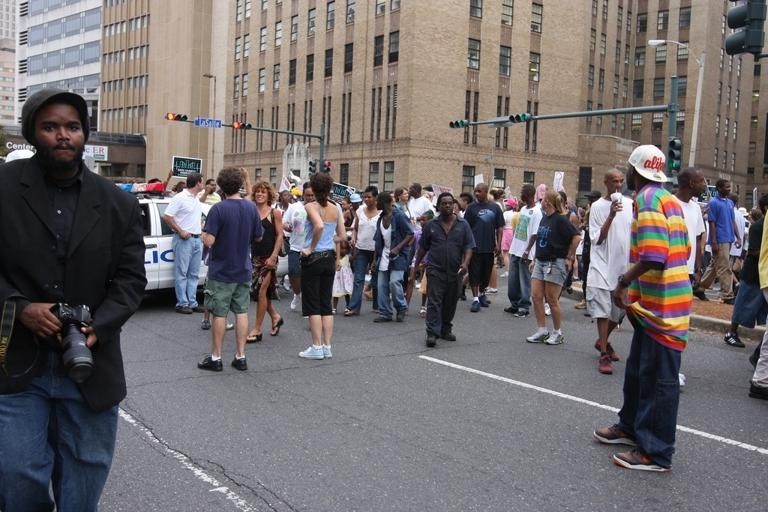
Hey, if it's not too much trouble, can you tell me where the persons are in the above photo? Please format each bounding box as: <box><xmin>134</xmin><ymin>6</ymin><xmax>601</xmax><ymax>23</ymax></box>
<box><xmin>585</xmin><ymin>145</ymin><xmax>768</xmax><ymax>474</ymax></box>
<box><xmin>337</xmin><ymin>184</ymin><xmax>602</xmax><ymax>345</ymax></box>
<box><xmin>1</xmin><ymin>89</ymin><xmax>144</xmax><ymax>511</ymax></box>
<box><xmin>148</xmin><ymin>165</ymin><xmax>347</xmax><ymax>371</ymax></box>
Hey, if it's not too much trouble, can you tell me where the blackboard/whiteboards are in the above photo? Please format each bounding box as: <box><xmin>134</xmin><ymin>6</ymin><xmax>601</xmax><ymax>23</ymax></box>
<box><xmin>333</xmin><ymin>182</ymin><xmax>355</xmax><ymax>197</ymax></box>
<box><xmin>171</xmin><ymin>156</ymin><xmax>202</xmax><ymax>179</ymax></box>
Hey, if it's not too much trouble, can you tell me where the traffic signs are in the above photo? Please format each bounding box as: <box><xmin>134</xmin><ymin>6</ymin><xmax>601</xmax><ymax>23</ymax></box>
<box><xmin>194</xmin><ymin>118</ymin><xmax>221</xmax><ymax>128</ymax></box>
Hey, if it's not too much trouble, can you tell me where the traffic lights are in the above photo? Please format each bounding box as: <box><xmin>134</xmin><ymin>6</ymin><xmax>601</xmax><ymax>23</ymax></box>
<box><xmin>449</xmin><ymin>119</ymin><xmax>468</xmax><ymax>129</ymax></box>
<box><xmin>308</xmin><ymin>161</ymin><xmax>316</xmax><ymax>177</ymax></box>
<box><xmin>724</xmin><ymin>0</ymin><xmax>746</xmax><ymax>58</ymax></box>
<box><xmin>669</xmin><ymin>136</ymin><xmax>680</xmax><ymax>174</ymax></box>
<box><xmin>167</xmin><ymin>113</ymin><xmax>187</xmax><ymax>121</ymax></box>
<box><xmin>510</xmin><ymin>112</ymin><xmax>530</xmax><ymax>124</ymax></box>
<box><xmin>323</xmin><ymin>160</ymin><xmax>331</xmax><ymax>173</ymax></box>
<box><xmin>232</xmin><ymin>122</ymin><xmax>251</xmax><ymax>131</ymax></box>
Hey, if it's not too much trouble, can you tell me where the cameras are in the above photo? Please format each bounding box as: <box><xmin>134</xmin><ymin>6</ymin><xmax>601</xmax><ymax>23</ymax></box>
<box><xmin>49</xmin><ymin>303</ymin><xmax>94</xmax><ymax>385</ymax></box>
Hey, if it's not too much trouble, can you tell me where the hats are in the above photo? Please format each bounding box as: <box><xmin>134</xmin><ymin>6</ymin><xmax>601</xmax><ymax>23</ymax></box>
<box><xmin>584</xmin><ymin>191</ymin><xmax>602</xmax><ymax>198</ymax></box>
<box><xmin>21</xmin><ymin>88</ymin><xmax>89</xmax><ymax>142</ymax></box>
<box><xmin>629</xmin><ymin>145</ymin><xmax>670</xmax><ymax>183</ymax></box>
<box><xmin>350</xmin><ymin>194</ymin><xmax>363</xmax><ymax>203</ymax></box>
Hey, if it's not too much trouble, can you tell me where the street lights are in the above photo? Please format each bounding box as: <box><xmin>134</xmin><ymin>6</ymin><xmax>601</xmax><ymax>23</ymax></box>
<box><xmin>644</xmin><ymin>38</ymin><xmax>709</xmax><ymax>167</ymax></box>
<box><xmin>203</xmin><ymin>73</ymin><xmax>217</xmax><ymax>181</ymax></box>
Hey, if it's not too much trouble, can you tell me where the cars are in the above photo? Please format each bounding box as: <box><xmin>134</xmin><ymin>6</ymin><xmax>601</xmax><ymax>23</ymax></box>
<box><xmin>112</xmin><ymin>180</ymin><xmax>291</xmax><ymax>301</ymax></box>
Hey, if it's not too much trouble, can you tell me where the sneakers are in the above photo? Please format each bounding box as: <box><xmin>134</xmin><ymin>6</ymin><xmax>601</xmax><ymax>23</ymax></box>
<box><xmin>693</xmin><ymin>283</ymin><xmax>741</xmax><ymax>305</ymax></box>
<box><xmin>612</xmin><ymin>446</ymin><xmax>673</xmax><ymax>472</ymax></box>
<box><xmin>299</xmin><ymin>345</ymin><xmax>332</xmax><ymax>359</ymax></box>
<box><xmin>594</xmin><ymin>339</ymin><xmax>621</xmax><ymax>361</ymax></box>
<box><xmin>724</xmin><ymin>332</ymin><xmax>745</xmax><ymax>346</ymax></box>
<box><xmin>426</xmin><ymin>334</ymin><xmax>437</xmax><ymax>347</ymax></box>
<box><xmin>460</xmin><ymin>270</ymin><xmax>573</xmax><ymax>318</ymax></box>
<box><xmin>440</xmin><ymin>330</ymin><xmax>456</xmax><ymax>341</ymax></box>
<box><xmin>575</xmin><ymin>298</ymin><xmax>588</xmax><ymax>309</ymax></box>
<box><xmin>175</xmin><ymin>306</ymin><xmax>283</xmax><ymax>343</ymax></box>
<box><xmin>593</xmin><ymin>425</ymin><xmax>635</xmax><ymax>446</ymax></box>
<box><xmin>364</xmin><ymin>287</ymin><xmax>426</xmax><ymax>323</ymax></box>
<box><xmin>290</xmin><ymin>293</ymin><xmax>360</xmax><ymax>316</ymax></box>
<box><xmin>527</xmin><ymin>332</ymin><xmax>550</xmax><ymax>343</ymax></box>
<box><xmin>600</xmin><ymin>352</ymin><xmax>613</xmax><ymax>374</ymax></box>
<box><xmin>747</xmin><ymin>384</ymin><xmax>768</xmax><ymax>399</ymax></box>
<box><xmin>544</xmin><ymin>334</ymin><xmax>567</xmax><ymax>345</ymax></box>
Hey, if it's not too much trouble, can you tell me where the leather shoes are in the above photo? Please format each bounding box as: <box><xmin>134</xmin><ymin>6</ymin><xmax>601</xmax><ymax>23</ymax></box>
<box><xmin>232</xmin><ymin>356</ymin><xmax>247</xmax><ymax>370</ymax></box>
<box><xmin>199</xmin><ymin>355</ymin><xmax>221</xmax><ymax>370</ymax></box>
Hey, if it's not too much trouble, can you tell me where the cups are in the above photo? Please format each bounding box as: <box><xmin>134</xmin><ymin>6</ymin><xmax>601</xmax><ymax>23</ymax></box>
<box><xmin>610</xmin><ymin>192</ymin><xmax>622</xmax><ymax>211</ymax></box>
